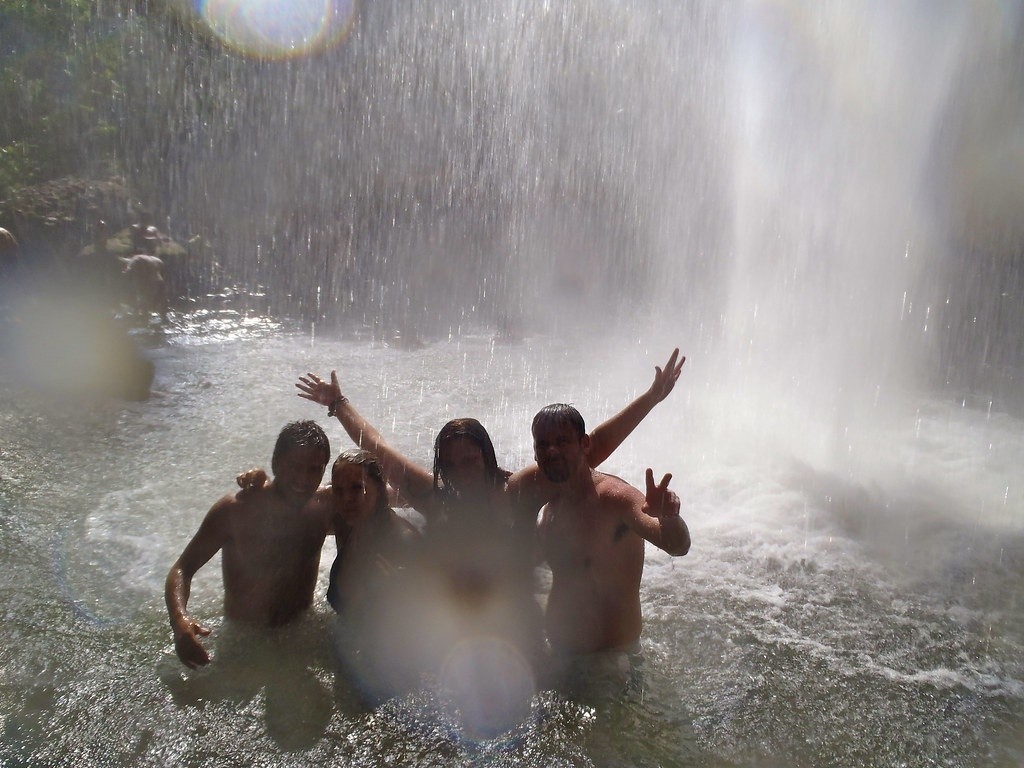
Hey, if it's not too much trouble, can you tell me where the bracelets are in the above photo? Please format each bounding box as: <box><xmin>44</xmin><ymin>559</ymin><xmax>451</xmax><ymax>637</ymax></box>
<box><xmin>327</xmin><ymin>395</ymin><xmax>350</xmax><ymax>417</ymax></box>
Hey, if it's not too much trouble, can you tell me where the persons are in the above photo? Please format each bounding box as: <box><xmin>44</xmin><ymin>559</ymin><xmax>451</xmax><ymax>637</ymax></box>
<box><xmin>507</xmin><ymin>404</ymin><xmax>690</xmax><ymax>657</ymax></box>
<box><xmin>72</xmin><ymin>213</ymin><xmax>168</xmax><ymax>314</ymax></box>
<box><xmin>165</xmin><ymin>418</ymin><xmax>513</xmax><ymax>670</ymax></box>
<box><xmin>237</xmin><ymin>449</ymin><xmax>449</xmax><ymax>632</ymax></box>
<box><xmin>295</xmin><ymin>348</ymin><xmax>686</xmax><ymax>655</ymax></box>
